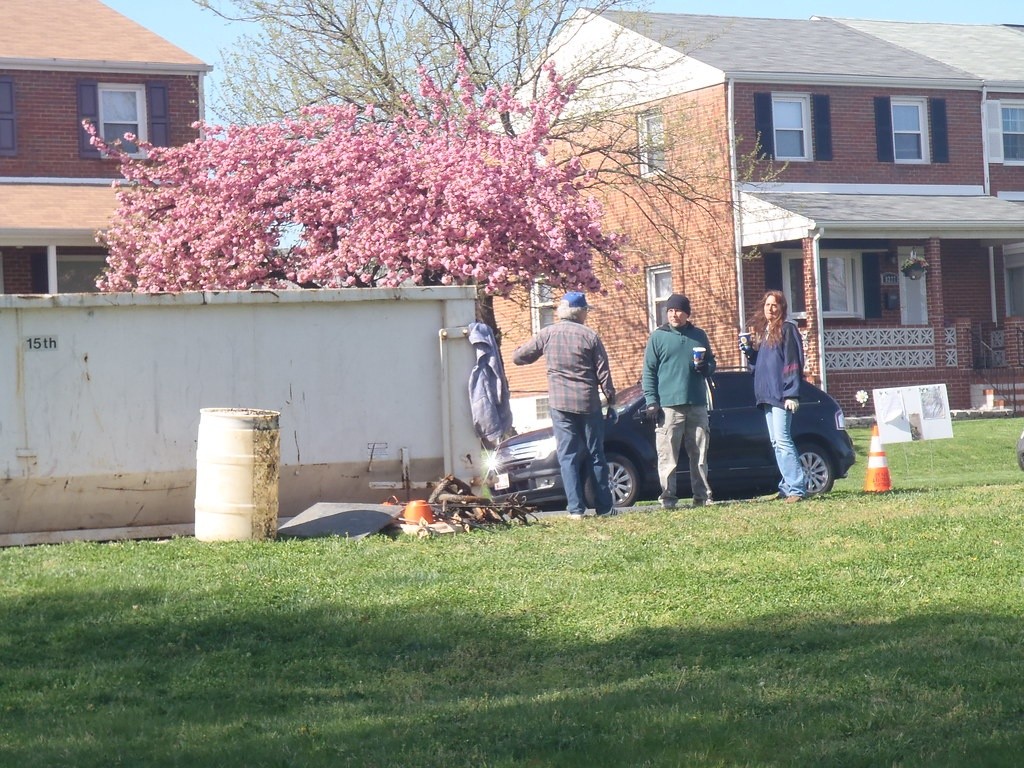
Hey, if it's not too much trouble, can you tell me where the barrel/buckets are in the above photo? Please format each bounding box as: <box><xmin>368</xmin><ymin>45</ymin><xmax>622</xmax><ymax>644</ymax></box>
<box><xmin>194</xmin><ymin>407</ymin><xmax>282</xmax><ymax>545</ymax></box>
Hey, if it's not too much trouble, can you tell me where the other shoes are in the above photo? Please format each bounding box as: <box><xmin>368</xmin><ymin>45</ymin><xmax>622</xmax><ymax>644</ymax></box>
<box><xmin>601</xmin><ymin>508</ymin><xmax>619</xmax><ymax>517</ymax></box>
<box><xmin>566</xmin><ymin>513</ymin><xmax>583</xmax><ymax>520</ymax></box>
<box><xmin>787</xmin><ymin>496</ymin><xmax>800</xmax><ymax>504</ymax></box>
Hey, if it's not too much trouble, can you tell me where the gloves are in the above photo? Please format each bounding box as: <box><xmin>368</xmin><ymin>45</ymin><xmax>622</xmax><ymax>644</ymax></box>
<box><xmin>645</xmin><ymin>403</ymin><xmax>663</xmax><ymax>424</ymax></box>
<box><xmin>738</xmin><ymin>339</ymin><xmax>755</xmax><ymax>354</ymax></box>
<box><xmin>690</xmin><ymin>361</ymin><xmax>710</xmax><ymax>377</ymax></box>
<box><xmin>605</xmin><ymin>404</ymin><xmax>618</xmax><ymax>424</ymax></box>
<box><xmin>785</xmin><ymin>398</ymin><xmax>800</xmax><ymax>414</ymax></box>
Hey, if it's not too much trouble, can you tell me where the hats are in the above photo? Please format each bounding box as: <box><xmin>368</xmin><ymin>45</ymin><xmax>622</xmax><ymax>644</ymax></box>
<box><xmin>561</xmin><ymin>291</ymin><xmax>592</xmax><ymax>307</ymax></box>
<box><xmin>666</xmin><ymin>294</ymin><xmax>690</xmax><ymax>316</ymax></box>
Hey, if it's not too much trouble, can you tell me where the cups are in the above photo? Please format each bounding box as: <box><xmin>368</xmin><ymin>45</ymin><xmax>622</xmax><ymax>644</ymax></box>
<box><xmin>738</xmin><ymin>334</ymin><xmax>751</xmax><ymax>350</ymax></box>
<box><xmin>693</xmin><ymin>347</ymin><xmax>706</xmax><ymax>365</ymax></box>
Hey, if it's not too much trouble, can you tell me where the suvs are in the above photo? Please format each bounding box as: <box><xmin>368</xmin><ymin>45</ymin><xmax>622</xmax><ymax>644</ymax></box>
<box><xmin>481</xmin><ymin>365</ymin><xmax>857</xmax><ymax>511</ymax></box>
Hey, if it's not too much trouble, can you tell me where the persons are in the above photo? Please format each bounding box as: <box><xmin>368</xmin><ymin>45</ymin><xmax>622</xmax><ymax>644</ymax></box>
<box><xmin>641</xmin><ymin>294</ymin><xmax>718</xmax><ymax>508</ymax></box>
<box><xmin>512</xmin><ymin>291</ymin><xmax>625</xmax><ymax>520</ymax></box>
<box><xmin>739</xmin><ymin>291</ymin><xmax>806</xmax><ymax>505</ymax></box>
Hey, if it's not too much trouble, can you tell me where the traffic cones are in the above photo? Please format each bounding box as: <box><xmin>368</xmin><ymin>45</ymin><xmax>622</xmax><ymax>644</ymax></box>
<box><xmin>858</xmin><ymin>425</ymin><xmax>893</xmax><ymax>492</ymax></box>
